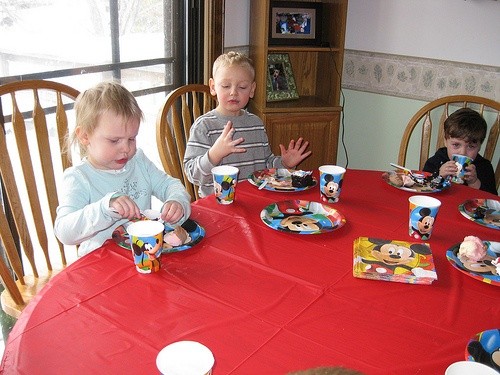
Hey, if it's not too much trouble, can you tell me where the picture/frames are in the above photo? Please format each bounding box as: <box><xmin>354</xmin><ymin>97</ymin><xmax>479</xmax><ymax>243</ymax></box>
<box><xmin>269</xmin><ymin>0</ymin><xmax>322</xmax><ymax>47</ymax></box>
<box><xmin>265</xmin><ymin>52</ymin><xmax>300</xmax><ymax>103</ymax></box>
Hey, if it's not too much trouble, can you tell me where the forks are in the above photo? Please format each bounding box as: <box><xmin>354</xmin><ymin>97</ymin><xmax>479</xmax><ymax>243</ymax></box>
<box><xmin>388</xmin><ymin>163</ymin><xmax>425</xmax><ymax>179</ymax></box>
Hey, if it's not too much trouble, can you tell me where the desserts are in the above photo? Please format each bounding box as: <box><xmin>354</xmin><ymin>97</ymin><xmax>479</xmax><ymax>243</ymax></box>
<box><xmin>471</xmin><ymin>206</ymin><xmax>487</xmax><ymax>219</ymax></box>
<box><xmin>459</xmin><ymin>235</ymin><xmax>488</xmax><ymax>261</ymax></box>
<box><xmin>162</xmin><ymin>225</ymin><xmax>188</xmax><ymax>247</ymax></box>
<box><xmin>290</xmin><ymin>169</ymin><xmax>315</xmax><ymax>188</ymax></box>
<box><xmin>388</xmin><ymin>173</ymin><xmax>415</xmax><ymax>187</ymax></box>
<box><xmin>430</xmin><ymin>176</ymin><xmax>444</xmax><ymax>190</ymax></box>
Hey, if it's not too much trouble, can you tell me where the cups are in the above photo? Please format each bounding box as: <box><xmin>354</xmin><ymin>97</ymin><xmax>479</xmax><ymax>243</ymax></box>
<box><xmin>408</xmin><ymin>194</ymin><xmax>442</xmax><ymax>240</ymax></box>
<box><xmin>210</xmin><ymin>164</ymin><xmax>240</xmax><ymax>205</ymax></box>
<box><xmin>155</xmin><ymin>340</ymin><xmax>214</xmax><ymax>374</ymax></box>
<box><xmin>446</xmin><ymin>155</ymin><xmax>473</xmax><ymax>184</ymax></box>
<box><xmin>444</xmin><ymin>360</ymin><xmax>499</xmax><ymax>375</ymax></box>
<box><xmin>126</xmin><ymin>219</ymin><xmax>165</xmax><ymax>273</ymax></box>
<box><xmin>317</xmin><ymin>164</ymin><xmax>346</xmax><ymax>204</ymax></box>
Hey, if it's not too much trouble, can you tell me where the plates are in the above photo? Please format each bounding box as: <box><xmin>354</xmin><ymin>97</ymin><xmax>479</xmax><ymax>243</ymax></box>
<box><xmin>457</xmin><ymin>198</ymin><xmax>500</xmax><ymax>231</ymax></box>
<box><xmin>382</xmin><ymin>168</ymin><xmax>451</xmax><ymax>193</ymax></box>
<box><xmin>111</xmin><ymin>213</ymin><xmax>206</xmax><ymax>252</ymax></box>
<box><xmin>247</xmin><ymin>168</ymin><xmax>319</xmax><ymax>191</ymax></box>
<box><xmin>445</xmin><ymin>239</ymin><xmax>500</xmax><ymax>289</ymax></box>
<box><xmin>465</xmin><ymin>328</ymin><xmax>500</xmax><ymax>374</ymax></box>
<box><xmin>259</xmin><ymin>200</ymin><xmax>346</xmax><ymax>234</ymax></box>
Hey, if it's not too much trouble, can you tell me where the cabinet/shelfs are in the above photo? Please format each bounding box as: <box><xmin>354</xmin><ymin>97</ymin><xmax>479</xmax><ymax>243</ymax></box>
<box><xmin>249</xmin><ymin>0</ymin><xmax>347</xmax><ymax>174</ymax></box>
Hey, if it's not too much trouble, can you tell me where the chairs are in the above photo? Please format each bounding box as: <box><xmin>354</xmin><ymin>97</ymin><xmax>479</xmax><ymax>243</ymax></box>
<box><xmin>157</xmin><ymin>85</ymin><xmax>223</xmax><ymax>207</ymax></box>
<box><xmin>397</xmin><ymin>94</ymin><xmax>500</xmax><ymax>196</ymax></box>
<box><xmin>0</xmin><ymin>79</ymin><xmax>80</xmax><ymax>319</ymax></box>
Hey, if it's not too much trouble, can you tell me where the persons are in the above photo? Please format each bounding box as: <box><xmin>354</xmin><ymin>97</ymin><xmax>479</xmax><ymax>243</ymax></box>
<box><xmin>54</xmin><ymin>79</ymin><xmax>192</xmax><ymax>256</ymax></box>
<box><xmin>183</xmin><ymin>51</ymin><xmax>311</xmax><ymax>199</ymax></box>
<box><xmin>276</xmin><ymin>13</ymin><xmax>309</xmax><ymax>34</ymax></box>
<box><xmin>423</xmin><ymin>108</ymin><xmax>498</xmax><ymax>196</ymax></box>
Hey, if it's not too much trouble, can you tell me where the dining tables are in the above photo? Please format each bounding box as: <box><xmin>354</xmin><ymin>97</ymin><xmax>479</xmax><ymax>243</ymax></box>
<box><xmin>1</xmin><ymin>166</ymin><xmax>500</xmax><ymax>375</ymax></box>
